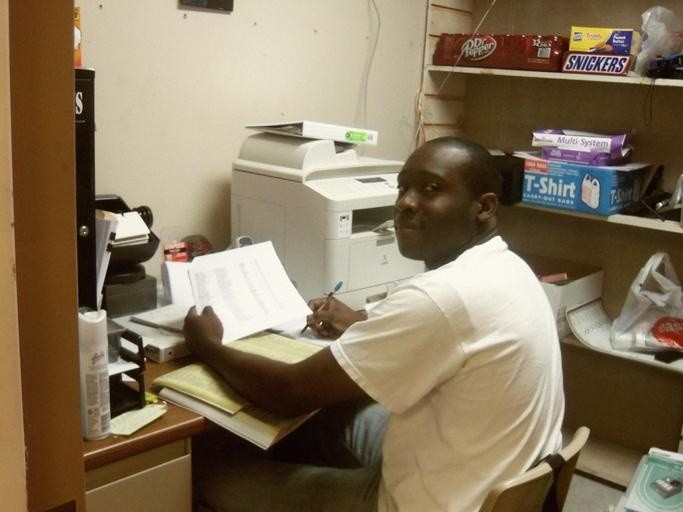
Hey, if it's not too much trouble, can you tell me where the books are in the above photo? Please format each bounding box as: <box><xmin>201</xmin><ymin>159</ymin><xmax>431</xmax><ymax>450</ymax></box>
<box><xmin>148</xmin><ymin>327</ymin><xmax>339</xmax><ymax>450</ymax></box>
<box><xmin>113</xmin><ymin>208</ymin><xmax>151</xmax><ymax>248</ymax></box>
<box><xmin>130</xmin><ymin>300</ymin><xmax>185</xmax><ymax>333</ymax></box>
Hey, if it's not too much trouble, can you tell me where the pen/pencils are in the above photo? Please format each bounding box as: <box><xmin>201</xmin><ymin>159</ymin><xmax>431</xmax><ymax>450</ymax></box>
<box><xmin>300</xmin><ymin>281</ymin><xmax>342</xmax><ymax>334</ymax></box>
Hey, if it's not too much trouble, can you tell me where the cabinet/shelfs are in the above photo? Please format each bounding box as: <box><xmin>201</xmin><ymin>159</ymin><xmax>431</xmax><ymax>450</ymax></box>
<box><xmin>426</xmin><ymin>3</ymin><xmax>682</xmax><ymax>491</ymax></box>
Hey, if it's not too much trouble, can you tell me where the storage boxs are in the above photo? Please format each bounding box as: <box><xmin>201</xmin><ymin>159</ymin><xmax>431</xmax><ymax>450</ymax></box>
<box><xmin>430</xmin><ymin>26</ymin><xmax>642</xmax><ymax>77</ymax></box>
<box><xmin>514</xmin><ymin>147</ymin><xmax>643</xmax><ymax>217</ymax></box>
<box><xmin>511</xmin><ymin>249</ymin><xmax>606</xmax><ymax>342</ymax></box>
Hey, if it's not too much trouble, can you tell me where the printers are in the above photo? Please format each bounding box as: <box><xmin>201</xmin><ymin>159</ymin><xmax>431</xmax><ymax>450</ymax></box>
<box><xmin>229</xmin><ymin>132</ymin><xmax>426</xmax><ymax>312</ymax></box>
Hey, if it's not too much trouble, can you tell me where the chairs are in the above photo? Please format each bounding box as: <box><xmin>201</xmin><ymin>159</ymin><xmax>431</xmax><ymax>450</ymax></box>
<box><xmin>480</xmin><ymin>425</ymin><xmax>591</xmax><ymax>512</ymax></box>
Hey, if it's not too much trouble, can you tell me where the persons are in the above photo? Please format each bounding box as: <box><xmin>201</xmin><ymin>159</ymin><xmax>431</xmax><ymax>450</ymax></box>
<box><xmin>183</xmin><ymin>135</ymin><xmax>567</xmax><ymax>511</ymax></box>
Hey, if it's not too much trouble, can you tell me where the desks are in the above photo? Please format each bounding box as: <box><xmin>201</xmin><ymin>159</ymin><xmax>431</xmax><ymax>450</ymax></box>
<box><xmin>83</xmin><ymin>347</ymin><xmax>214</xmax><ymax>512</ymax></box>
